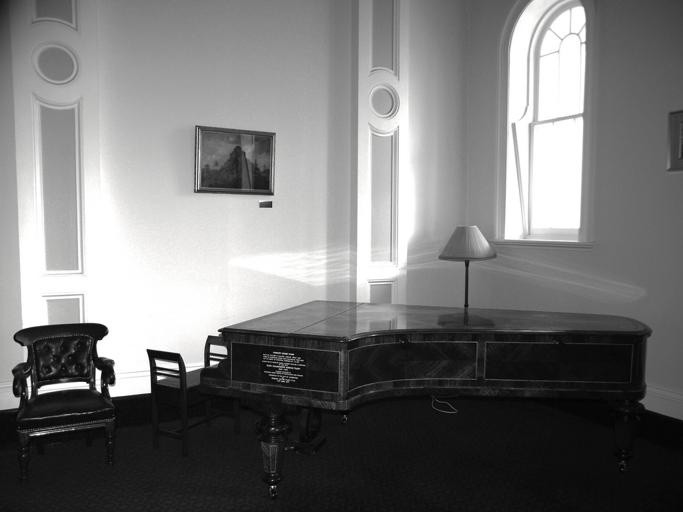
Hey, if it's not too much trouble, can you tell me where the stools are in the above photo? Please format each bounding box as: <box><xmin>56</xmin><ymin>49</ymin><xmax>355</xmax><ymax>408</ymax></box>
<box><xmin>143</xmin><ymin>336</ymin><xmax>243</xmax><ymax>461</ymax></box>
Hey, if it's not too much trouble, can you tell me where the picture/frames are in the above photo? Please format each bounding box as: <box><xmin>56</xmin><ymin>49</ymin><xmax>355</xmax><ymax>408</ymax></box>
<box><xmin>663</xmin><ymin>109</ymin><xmax>683</xmax><ymax>174</ymax></box>
<box><xmin>191</xmin><ymin>122</ymin><xmax>276</xmax><ymax>196</ymax></box>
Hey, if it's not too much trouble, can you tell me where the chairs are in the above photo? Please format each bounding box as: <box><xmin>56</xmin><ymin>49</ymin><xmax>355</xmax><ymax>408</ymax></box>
<box><xmin>8</xmin><ymin>322</ymin><xmax>123</xmax><ymax>483</ymax></box>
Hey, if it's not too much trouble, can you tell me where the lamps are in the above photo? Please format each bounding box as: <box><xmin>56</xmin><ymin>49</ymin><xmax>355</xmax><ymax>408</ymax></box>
<box><xmin>436</xmin><ymin>223</ymin><xmax>504</xmax><ymax>329</ymax></box>
